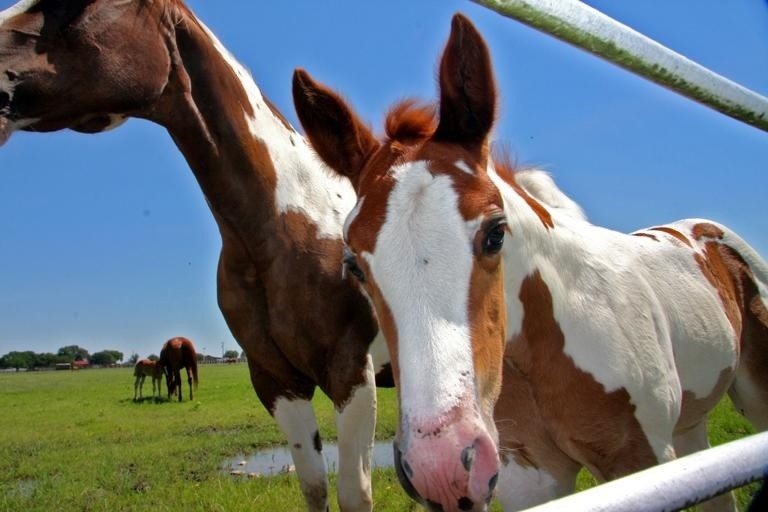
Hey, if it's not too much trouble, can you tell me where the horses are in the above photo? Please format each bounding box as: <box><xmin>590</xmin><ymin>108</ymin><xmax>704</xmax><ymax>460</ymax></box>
<box><xmin>290</xmin><ymin>9</ymin><xmax>768</xmax><ymax>512</ymax></box>
<box><xmin>133</xmin><ymin>358</ymin><xmax>164</xmax><ymax>402</ymax></box>
<box><xmin>0</xmin><ymin>0</ymin><xmax>589</xmax><ymax>512</ymax></box>
<box><xmin>160</xmin><ymin>336</ymin><xmax>199</xmax><ymax>402</ymax></box>
